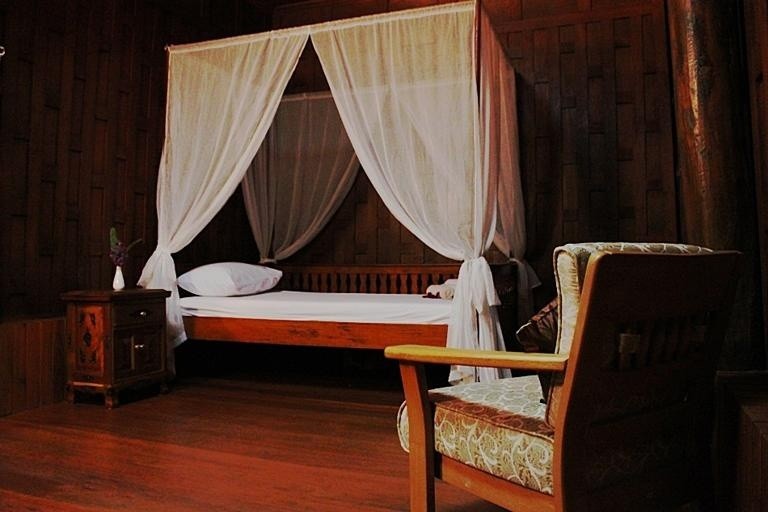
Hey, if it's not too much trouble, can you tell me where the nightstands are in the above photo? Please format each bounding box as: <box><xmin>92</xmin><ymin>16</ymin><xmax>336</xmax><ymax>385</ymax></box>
<box><xmin>59</xmin><ymin>289</ymin><xmax>171</xmax><ymax>411</ymax></box>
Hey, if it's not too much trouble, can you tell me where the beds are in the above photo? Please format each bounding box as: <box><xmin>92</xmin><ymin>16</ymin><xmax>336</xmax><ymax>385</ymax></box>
<box><xmin>135</xmin><ymin>0</ymin><xmax>541</xmax><ymax>385</ymax></box>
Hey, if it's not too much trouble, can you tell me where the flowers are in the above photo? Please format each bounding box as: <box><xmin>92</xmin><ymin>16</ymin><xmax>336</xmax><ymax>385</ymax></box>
<box><xmin>108</xmin><ymin>223</ymin><xmax>144</xmax><ymax>266</ymax></box>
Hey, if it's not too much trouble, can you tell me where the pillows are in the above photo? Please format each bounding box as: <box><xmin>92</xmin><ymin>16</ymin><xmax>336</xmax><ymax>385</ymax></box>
<box><xmin>542</xmin><ymin>242</ymin><xmax>714</xmax><ymax>431</ymax></box>
<box><xmin>178</xmin><ymin>262</ymin><xmax>284</xmax><ymax>297</ymax></box>
<box><xmin>515</xmin><ymin>297</ymin><xmax>558</xmax><ymax>403</ymax></box>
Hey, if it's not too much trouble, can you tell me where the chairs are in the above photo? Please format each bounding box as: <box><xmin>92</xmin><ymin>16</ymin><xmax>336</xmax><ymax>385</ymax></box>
<box><xmin>383</xmin><ymin>250</ymin><xmax>744</xmax><ymax>512</ymax></box>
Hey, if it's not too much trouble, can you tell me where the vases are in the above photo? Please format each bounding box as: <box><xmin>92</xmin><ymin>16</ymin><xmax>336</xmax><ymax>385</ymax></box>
<box><xmin>113</xmin><ymin>265</ymin><xmax>126</xmax><ymax>292</ymax></box>
